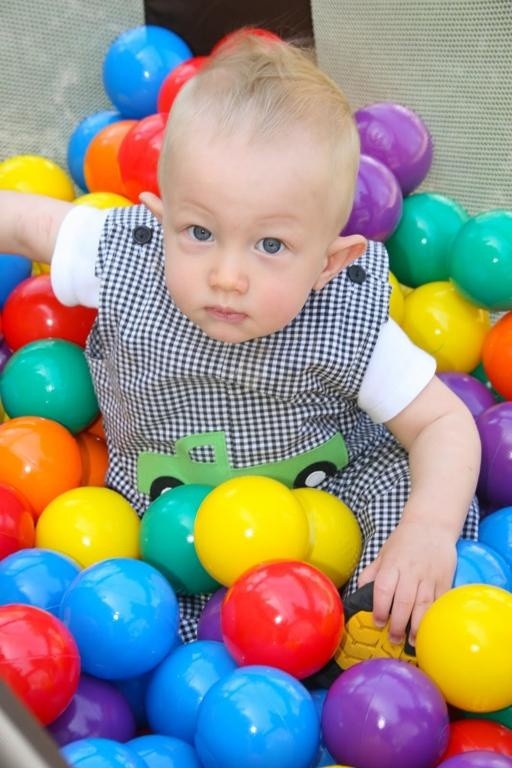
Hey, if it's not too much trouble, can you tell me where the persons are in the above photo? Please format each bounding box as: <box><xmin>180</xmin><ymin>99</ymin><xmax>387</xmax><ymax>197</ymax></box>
<box><xmin>1</xmin><ymin>34</ymin><xmax>482</xmax><ymax>693</ymax></box>
